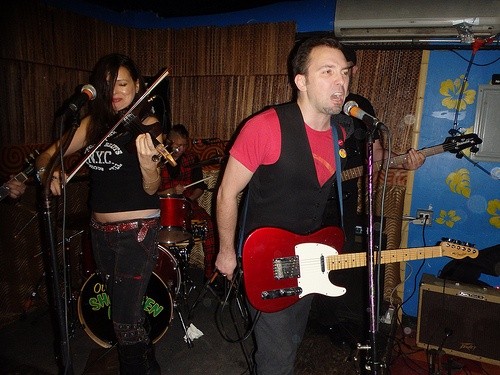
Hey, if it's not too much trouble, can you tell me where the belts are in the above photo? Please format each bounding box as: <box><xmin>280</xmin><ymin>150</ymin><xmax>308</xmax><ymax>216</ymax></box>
<box><xmin>90</xmin><ymin>219</ymin><xmax>155</xmax><ymax>242</ymax></box>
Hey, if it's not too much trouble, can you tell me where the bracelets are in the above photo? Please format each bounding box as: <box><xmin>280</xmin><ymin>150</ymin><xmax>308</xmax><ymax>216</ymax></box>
<box><xmin>36</xmin><ymin>166</ymin><xmax>45</xmax><ymax>181</ymax></box>
<box><xmin>143</xmin><ymin>169</ymin><xmax>160</xmax><ymax>183</ymax></box>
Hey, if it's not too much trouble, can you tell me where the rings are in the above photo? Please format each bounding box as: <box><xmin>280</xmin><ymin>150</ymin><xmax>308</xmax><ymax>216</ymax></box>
<box><xmin>222</xmin><ymin>274</ymin><xmax>226</xmax><ymax>276</ymax></box>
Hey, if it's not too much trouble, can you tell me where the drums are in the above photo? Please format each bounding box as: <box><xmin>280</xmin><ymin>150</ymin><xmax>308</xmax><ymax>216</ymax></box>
<box><xmin>157</xmin><ymin>194</ymin><xmax>192</xmax><ymax>244</ymax></box>
<box><xmin>77</xmin><ymin>244</ymin><xmax>181</xmax><ymax>348</ymax></box>
<box><xmin>182</xmin><ymin>218</ymin><xmax>208</xmax><ymax>243</ymax></box>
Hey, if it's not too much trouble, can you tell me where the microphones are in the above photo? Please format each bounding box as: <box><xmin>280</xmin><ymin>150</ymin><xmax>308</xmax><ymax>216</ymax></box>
<box><xmin>68</xmin><ymin>84</ymin><xmax>96</xmax><ymax>112</ymax></box>
<box><xmin>343</xmin><ymin>101</ymin><xmax>388</xmax><ymax>131</ymax></box>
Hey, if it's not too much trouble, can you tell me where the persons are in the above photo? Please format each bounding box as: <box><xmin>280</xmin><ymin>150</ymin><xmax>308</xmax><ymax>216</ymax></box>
<box><xmin>161</xmin><ymin>124</ymin><xmax>217</xmax><ymax>287</ymax></box>
<box><xmin>6</xmin><ymin>56</ymin><xmax>163</xmax><ymax>375</ymax></box>
<box><xmin>215</xmin><ymin>39</ymin><xmax>426</xmax><ymax>375</ymax></box>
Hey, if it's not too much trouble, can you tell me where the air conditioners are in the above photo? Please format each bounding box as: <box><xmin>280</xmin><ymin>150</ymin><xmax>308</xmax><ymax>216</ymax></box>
<box><xmin>334</xmin><ymin>0</ymin><xmax>500</xmax><ymax>43</ymax></box>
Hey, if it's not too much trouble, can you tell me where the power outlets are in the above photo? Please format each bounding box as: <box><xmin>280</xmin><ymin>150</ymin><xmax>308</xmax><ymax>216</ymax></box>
<box><xmin>416</xmin><ymin>211</ymin><xmax>432</xmax><ymax>226</ymax></box>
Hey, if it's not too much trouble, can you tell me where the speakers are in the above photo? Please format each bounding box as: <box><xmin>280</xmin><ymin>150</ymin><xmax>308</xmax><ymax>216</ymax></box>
<box><xmin>415</xmin><ymin>274</ymin><xmax>500</xmax><ymax>366</ymax></box>
<box><xmin>0</xmin><ymin>172</ymin><xmax>93</xmax><ymax>327</ymax></box>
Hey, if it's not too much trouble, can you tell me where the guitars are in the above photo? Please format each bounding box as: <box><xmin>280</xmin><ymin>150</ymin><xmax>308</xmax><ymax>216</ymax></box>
<box><xmin>340</xmin><ymin>126</ymin><xmax>483</xmax><ymax>182</ymax></box>
<box><xmin>242</xmin><ymin>226</ymin><xmax>479</xmax><ymax>314</ymax></box>
<box><xmin>1</xmin><ymin>148</ymin><xmax>40</xmax><ymax>201</ymax></box>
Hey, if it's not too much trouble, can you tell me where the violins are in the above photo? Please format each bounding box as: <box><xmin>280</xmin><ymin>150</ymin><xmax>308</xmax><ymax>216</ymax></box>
<box><xmin>109</xmin><ymin>90</ymin><xmax>182</xmax><ymax>167</ymax></box>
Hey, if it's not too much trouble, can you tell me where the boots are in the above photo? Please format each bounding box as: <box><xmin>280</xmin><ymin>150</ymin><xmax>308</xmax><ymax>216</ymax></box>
<box><xmin>112</xmin><ymin>318</ymin><xmax>160</xmax><ymax>375</ymax></box>
